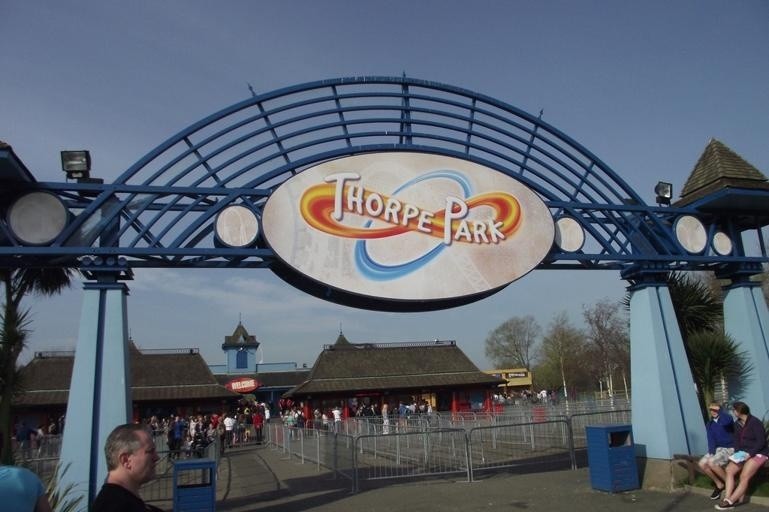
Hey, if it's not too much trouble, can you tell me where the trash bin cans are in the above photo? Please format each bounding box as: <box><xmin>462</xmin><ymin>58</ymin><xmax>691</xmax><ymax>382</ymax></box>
<box><xmin>585</xmin><ymin>423</ymin><xmax>641</xmax><ymax>493</ymax></box>
<box><xmin>172</xmin><ymin>457</ymin><xmax>217</xmax><ymax>512</ymax></box>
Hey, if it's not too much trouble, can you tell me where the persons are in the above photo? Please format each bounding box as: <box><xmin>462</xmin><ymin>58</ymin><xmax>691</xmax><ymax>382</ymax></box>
<box><xmin>713</xmin><ymin>402</ymin><xmax>768</xmax><ymax>510</ymax></box>
<box><xmin>697</xmin><ymin>403</ymin><xmax>734</xmax><ymax>501</ymax></box>
<box><xmin>15</xmin><ymin>407</ymin><xmax>66</xmax><ymax>464</ymax></box>
<box><xmin>0</xmin><ymin>464</ymin><xmax>58</xmax><ymax>512</ymax></box>
<box><xmin>491</xmin><ymin>387</ymin><xmax>557</xmax><ymax>410</ymax></box>
<box><xmin>143</xmin><ymin>402</ymin><xmax>270</xmax><ymax>463</ymax></box>
<box><xmin>279</xmin><ymin>405</ymin><xmax>343</xmax><ymax>441</ymax></box>
<box><xmin>352</xmin><ymin>400</ymin><xmax>433</xmax><ymax>434</ymax></box>
<box><xmin>90</xmin><ymin>422</ymin><xmax>160</xmax><ymax>512</ymax></box>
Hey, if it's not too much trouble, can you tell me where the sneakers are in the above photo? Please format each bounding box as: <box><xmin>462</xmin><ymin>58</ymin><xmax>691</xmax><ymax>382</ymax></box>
<box><xmin>710</xmin><ymin>486</ymin><xmax>736</xmax><ymax>509</ymax></box>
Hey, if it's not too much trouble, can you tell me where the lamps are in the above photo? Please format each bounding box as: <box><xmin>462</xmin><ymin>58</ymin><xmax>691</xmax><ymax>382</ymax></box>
<box><xmin>61</xmin><ymin>149</ymin><xmax>91</xmax><ymax>183</ymax></box>
<box><xmin>654</xmin><ymin>181</ymin><xmax>673</xmax><ymax>206</ymax></box>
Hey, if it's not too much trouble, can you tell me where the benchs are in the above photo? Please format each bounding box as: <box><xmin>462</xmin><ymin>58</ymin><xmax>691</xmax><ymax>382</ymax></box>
<box><xmin>669</xmin><ymin>454</ymin><xmax>769</xmax><ymax>491</ymax></box>
